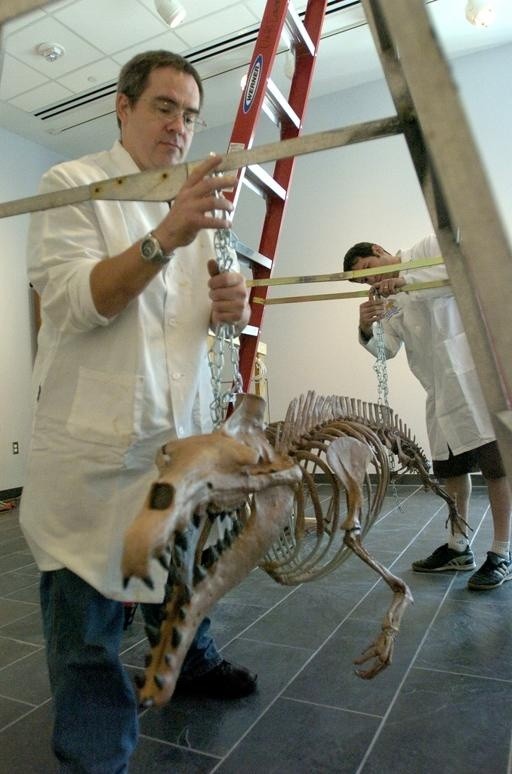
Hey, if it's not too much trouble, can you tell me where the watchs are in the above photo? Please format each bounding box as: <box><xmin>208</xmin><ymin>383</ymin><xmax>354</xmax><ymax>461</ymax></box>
<box><xmin>139</xmin><ymin>229</ymin><xmax>175</xmax><ymax>266</ymax></box>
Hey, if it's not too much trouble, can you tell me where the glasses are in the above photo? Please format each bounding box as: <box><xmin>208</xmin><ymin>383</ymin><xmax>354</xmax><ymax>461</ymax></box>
<box><xmin>134</xmin><ymin>93</ymin><xmax>208</xmax><ymax>134</ymax></box>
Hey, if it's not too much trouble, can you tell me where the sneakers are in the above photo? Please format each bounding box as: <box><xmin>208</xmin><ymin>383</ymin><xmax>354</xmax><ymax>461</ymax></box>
<box><xmin>466</xmin><ymin>550</ymin><xmax>512</xmax><ymax>593</ymax></box>
<box><xmin>147</xmin><ymin>656</ymin><xmax>258</xmax><ymax>701</ymax></box>
<box><xmin>410</xmin><ymin>542</ymin><xmax>476</xmax><ymax>573</ymax></box>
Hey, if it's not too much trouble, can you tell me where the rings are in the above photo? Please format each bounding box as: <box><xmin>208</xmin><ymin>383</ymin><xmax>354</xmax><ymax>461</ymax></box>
<box><xmin>384</xmin><ymin>285</ymin><xmax>389</xmax><ymax>289</ymax></box>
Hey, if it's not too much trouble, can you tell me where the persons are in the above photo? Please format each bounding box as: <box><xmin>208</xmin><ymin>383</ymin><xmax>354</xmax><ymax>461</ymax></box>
<box><xmin>343</xmin><ymin>231</ymin><xmax>512</xmax><ymax>590</ymax></box>
<box><xmin>20</xmin><ymin>49</ymin><xmax>259</xmax><ymax>774</ymax></box>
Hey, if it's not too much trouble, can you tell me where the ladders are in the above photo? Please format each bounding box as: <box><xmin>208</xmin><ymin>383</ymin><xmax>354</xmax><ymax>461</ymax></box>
<box><xmin>217</xmin><ymin>0</ymin><xmax>325</xmax><ymax>424</ymax></box>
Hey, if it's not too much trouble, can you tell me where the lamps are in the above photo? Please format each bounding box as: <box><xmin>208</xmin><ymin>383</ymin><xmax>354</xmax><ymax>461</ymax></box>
<box><xmin>155</xmin><ymin>0</ymin><xmax>186</xmax><ymax>28</ymax></box>
